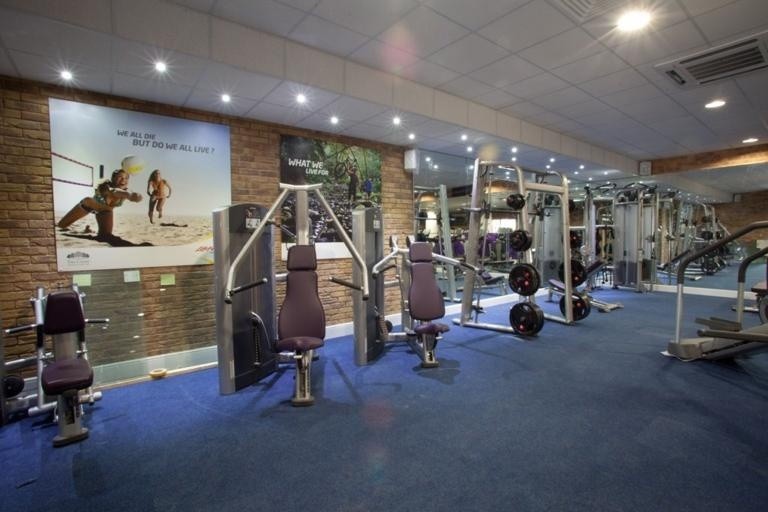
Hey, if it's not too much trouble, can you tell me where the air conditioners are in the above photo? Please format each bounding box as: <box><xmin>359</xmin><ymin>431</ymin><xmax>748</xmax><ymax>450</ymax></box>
<box><xmin>654</xmin><ymin>29</ymin><xmax>768</xmax><ymax>90</ymax></box>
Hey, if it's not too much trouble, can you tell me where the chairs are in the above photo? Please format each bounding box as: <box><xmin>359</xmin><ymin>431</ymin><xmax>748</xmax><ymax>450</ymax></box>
<box><xmin>409</xmin><ymin>242</ymin><xmax>450</xmax><ymax>368</ymax></box>
<box><xmin>40</xmin><ymin>290</ymin><xmax>94</xmax><ymax>448</ymax></box>
<box><xmin>274</xmin><ymin>245</ymin><xmax>326</xmax><ymax>407</ymax></box>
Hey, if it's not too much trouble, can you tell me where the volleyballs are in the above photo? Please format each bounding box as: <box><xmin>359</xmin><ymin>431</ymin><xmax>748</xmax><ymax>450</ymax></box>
<box><xmin>121</xmin><ymin>156</ymin><xmax>143</xmax><ymax>174</ymax></box>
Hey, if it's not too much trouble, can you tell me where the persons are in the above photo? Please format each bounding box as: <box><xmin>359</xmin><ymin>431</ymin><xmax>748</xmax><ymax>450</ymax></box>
<box><xmin>59</xmin><ymin>169</ymin><xmax>143</xmax><ymax>238</ymax></box>
<box><xmin>147</xmin><ymin>170</ymin><xmax>171</xmax><ymax>222</ymax></box>
<box><xmin>362</xmin><ymin>177</ymin><xmax>374</xmax><ymax>203</ymax></box>
<box><xmin>417</xmin><ymin>212</ymin><xmax>428</xmax><ymax>230</ymax></box>
<box><xmin>345</xmin><ymin>168</ymin><xmax>358</xmax><ymax>202</ymax></box>
